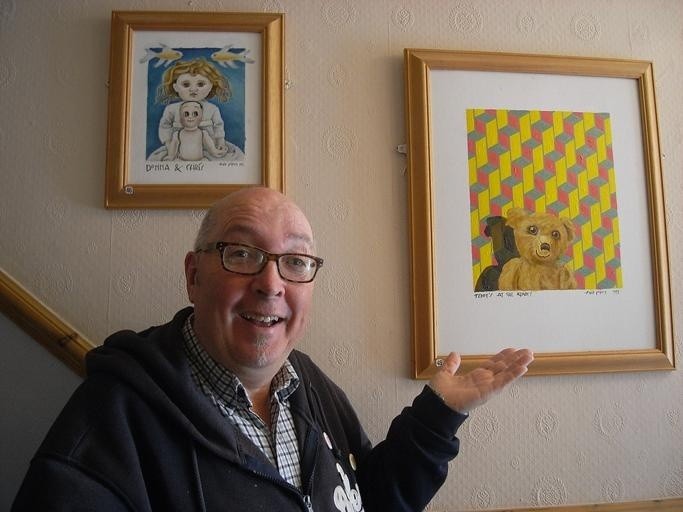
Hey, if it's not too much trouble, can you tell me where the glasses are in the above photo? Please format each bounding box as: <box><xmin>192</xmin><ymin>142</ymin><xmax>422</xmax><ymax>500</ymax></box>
<box><xmin>191</xmin><ymin>240</ymin><xmax>323</xmax><ymax>283</ymax></box>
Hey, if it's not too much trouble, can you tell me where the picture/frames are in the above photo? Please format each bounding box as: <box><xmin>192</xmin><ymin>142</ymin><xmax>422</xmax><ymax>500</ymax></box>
<box><xmin>104</xmin><ymin>9</ymin><xmax>285</xmax><ymax>212</ymax></box>
<box><xmin>402</xmin><ymin>47</ymin><xmax>675</xmax><ymax>381</ymax></box>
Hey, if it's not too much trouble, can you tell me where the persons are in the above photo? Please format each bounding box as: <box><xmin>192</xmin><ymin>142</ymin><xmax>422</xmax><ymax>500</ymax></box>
<box><xmin>145</xmin><ymin>63</ymin><xmax>245</xmax><ymax>161</ymax></box>
<box><xmin>166</xmin><ymin>100</ymin><xmax>220</xmax><ymax>161</ymax></box>
<box><xmin>10</xmin><ymin>186</ymin><xmax>534</xmax><ymax>511</ymax></box>
